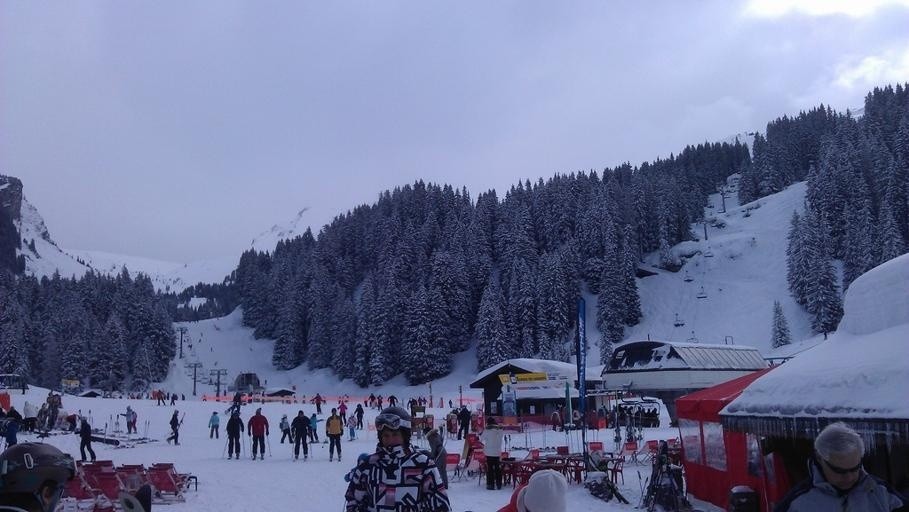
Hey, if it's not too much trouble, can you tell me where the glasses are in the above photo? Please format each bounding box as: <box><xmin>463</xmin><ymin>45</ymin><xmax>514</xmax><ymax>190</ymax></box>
<box><xmin>819</xmin><ymin>456</ymin><xmax>863</xmax><ymax>474</ymax></box>
<box><xmin>375</xmin><ymin>414</ymin><xmax>401</xmax><ymax>431</ymax></box>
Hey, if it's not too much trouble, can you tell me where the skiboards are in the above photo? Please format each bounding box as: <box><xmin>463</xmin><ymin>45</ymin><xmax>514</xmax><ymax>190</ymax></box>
<box><xmin>645</xmin><ymin>440</ymin><xmax>691</xmax><ymax>512</ymax></box>
<box><xmin>588</xmin><ymin>455</ymin><xmax>629</xmax><ymax>504</ymax></box>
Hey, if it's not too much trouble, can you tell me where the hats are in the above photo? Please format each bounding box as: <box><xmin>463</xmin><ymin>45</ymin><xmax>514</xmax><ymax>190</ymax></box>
<box><xmin>523</xmin><ymin>468</ymin><xmax>570</xmax><ymax>512</ymax></box>
<box><xmin>256</xmin><ymin>407</ymin><xmax>262</xmax><ymax>412</ymax></box>
<box><xmin>233</xmin><ymin>410</ymin><xmax>241</xmax><ymax>416</ymax></box>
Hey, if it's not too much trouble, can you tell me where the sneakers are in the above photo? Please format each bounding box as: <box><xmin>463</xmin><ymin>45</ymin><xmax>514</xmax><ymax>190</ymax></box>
<box><xmin>23</xmin><ymin>430</ymin><xmax>35</xmax><ymax>435</ymax></box>
<box><xmin>166</xmin><ymin>438</ymin><xmax>342</xmax><ymax>462</ymax></box>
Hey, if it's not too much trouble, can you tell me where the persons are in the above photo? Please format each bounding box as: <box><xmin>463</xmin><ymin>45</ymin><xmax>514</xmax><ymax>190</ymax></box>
<box><xmin>478</xmin><ymin>417</ymin><xmax>504</xmax><ymax>490</ymax></box>
<box><xmin>169</xmin><ymin>394</ymin><xmax>175</xmax><ymax>406</ymax></box>
<box><xmin>607</xmin><ymin>400</ymin><xmax>662</xmax><ymax>432</ymax></box>
<box><xmin>35</xmin><ymin>403</ymin><xmax>49</xmax><ymax>432</ymax></box>
<box><xmin>344</xmin><ymin>453</ymin><xmax>369</xmax><ymax>482</ymax></box>
<box><xmin>119</xmin><ymin>406</ymin><xmax>137</xmax><ymax>433</ymax></box>
<box><xmin>771</xmin><ymin>416</ymin><xmax>909</xmax><ymax>511</ymax></box>
<box><xmin>23</xmin><ymin>401</ymin><xmax>37</xmax><ymax>436</ymax></box>
<box><xmin>420</xmin><ymin>426</ymin><xmax>450</xmax><ymax>492</ymax></box>
<box><xmin>497</xmin><ymin>468</ymin><xmax>570</xmax><ymax>512</ymax></box>
<box><xmin>208</xmin><ymin>410</ymin><xmax>219</xmax><ymax>440</ymax></box>
<box><xmin>166</xmin><ymin>410</ymin><xmax>181</xmax><ymax>446</ymax></box>
<box><xmin>345</xmin><ymin>405</ymin><xmax>453</xmax><ymax>512</ymax></box>
<box><xmin>79</xmin><ymin>417</ymin><xmax>97</xmax><ymax>463</ymax></box>
<box><xmin>156</xmin><ymin>390</ymin><xmax>166</xmax><ymax>406</ymax></box>
<box><xmin>224</xmin><ymin>391</ymin><xmax>472</xmax><ymax>461</ymax></box>
<box><xmin>0</xmin><ymin>441</ymin><xmax>78</xmax><ymax>511</ymax></box>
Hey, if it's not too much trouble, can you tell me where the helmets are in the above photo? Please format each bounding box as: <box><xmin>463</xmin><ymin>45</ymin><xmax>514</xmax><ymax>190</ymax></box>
<box><xmin>374</xmin><ymin>405</ymin><xmax>412</xmax><ymax>432</ymax></box>
<box><xmin>0</xmin><ymin>441</ymin><xmax>76</xmax><ymax>494</ymax></box>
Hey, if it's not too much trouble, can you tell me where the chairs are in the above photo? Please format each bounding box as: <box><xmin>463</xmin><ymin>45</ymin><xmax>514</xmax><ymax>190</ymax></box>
<box><xmin>446</xmin><ymin>434</ymin><xmax>699</xmax><ymax>490</ymax></box>
<box><xmin>61</xmin><ymin>460</ymin><xmax>191</xmax><ymax>508</ymax></box>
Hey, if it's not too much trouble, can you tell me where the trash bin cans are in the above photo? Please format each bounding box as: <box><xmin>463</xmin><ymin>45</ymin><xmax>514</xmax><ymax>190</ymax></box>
<box><xmin>730</xmin><ymin>486</ymin><xmax>759</xmax><ymax>512</ymax></box>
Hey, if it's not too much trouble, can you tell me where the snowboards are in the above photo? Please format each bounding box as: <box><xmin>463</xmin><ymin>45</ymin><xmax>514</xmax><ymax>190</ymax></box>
<box><xmin>90</xmin><ymin>436</ymin><xmax>119</xmax><ymax>445</ymax></box>
